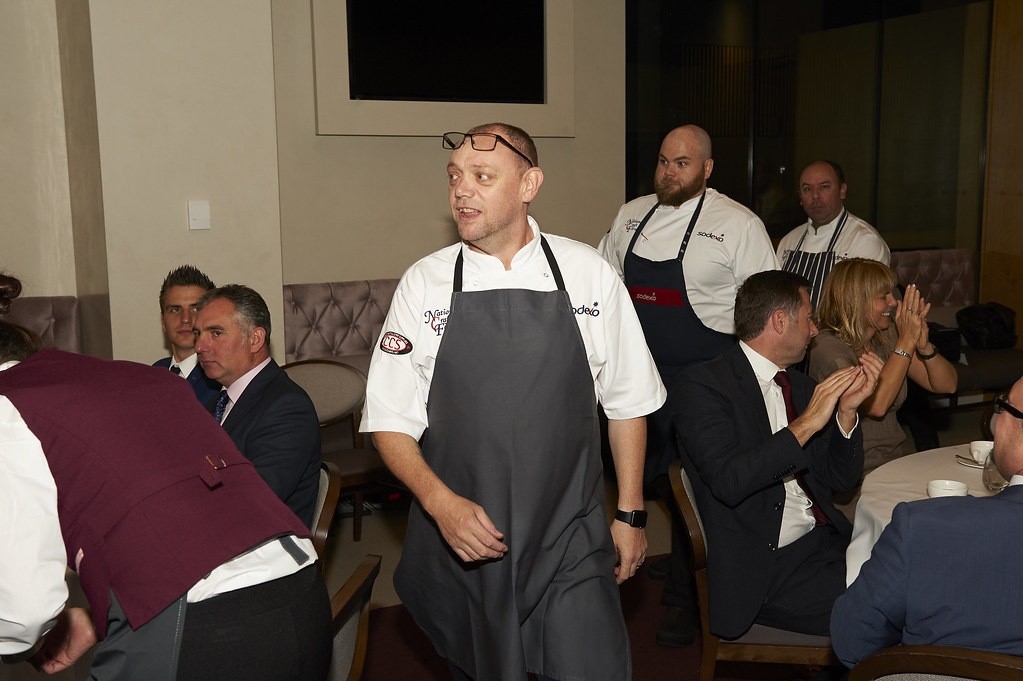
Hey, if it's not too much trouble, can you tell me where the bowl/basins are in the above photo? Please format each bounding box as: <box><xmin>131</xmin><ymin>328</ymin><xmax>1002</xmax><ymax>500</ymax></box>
<box><xmin>969</xmin><ymin>440</ymin><xmax>995</xmax><ymax>466</ymax></box>
<box><xmin>927</xmin><ymin>479</ymin><xmax>969</xmax><ymax>499</ymax></box>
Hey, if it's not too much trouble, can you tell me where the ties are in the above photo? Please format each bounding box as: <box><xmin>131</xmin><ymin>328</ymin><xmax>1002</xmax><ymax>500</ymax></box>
<box><xmin>773</xmin><ymin>369</ymin><xmax>829</xmax><ymax>526</ymax></box>
<box><xmin>212</xmin><ymin>389</ymin><xmax>230</xmax><ymax>425</ymax></box>
<box><xmin>170</xmin><ymin>365</ymin><xmax>181</xmax><ymax>374</ymax></box>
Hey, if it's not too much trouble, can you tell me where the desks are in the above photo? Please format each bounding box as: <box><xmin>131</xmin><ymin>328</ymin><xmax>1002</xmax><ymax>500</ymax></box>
<box><xmin>844</xmin><ymin>442</ymin><xmax>1012</xmax><ymax>593</ymax></box>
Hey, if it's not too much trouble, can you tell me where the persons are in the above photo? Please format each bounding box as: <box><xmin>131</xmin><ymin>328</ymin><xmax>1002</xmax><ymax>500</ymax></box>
<box><xmin>805</xmin><ymin>256</ymin><xmax>957</xmax><ymax>501</ymax></box>
<box><xmin>0</xmin><ymin>264</ymin><xmax>335</xmax><ymax>681</ymax></box>
<box><xmin>357</xmin><ymin>122</ymin><xmax>668</xmax><ymax>681</ymax></box>
<box><xmin>773</xmin><ymin>160</ymin><xmax>891</xmax><ymax>327</ymax></box>
<box><xmin>598</xmin><ymin>124</ymin><xmax>780</xmax><ymax>641</ymax></box>
<box><xmin>671</xmin><ymin>270</ymin><xmax>854</xmax><ymax>639</ymax></box>
<box><xmin>828</xmin><ymin>372</ymin><xmax>1023</xmax><ymax>667</ymax></box>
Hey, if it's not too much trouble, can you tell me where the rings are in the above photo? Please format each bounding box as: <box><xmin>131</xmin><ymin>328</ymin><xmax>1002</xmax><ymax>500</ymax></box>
<box><xmin>637</xmin><ymin>564</ymin><xmax>642</xmax><ymax>567</ymax></box>
<box><xmin>907</xmin><ymin>307</ymin><xmax>911</xmax><ymax>312</ymax></box>
<box><xmin>912</xmin><ymin>311</ymin><xmax>920</xmax><ymax>315</ymax></box>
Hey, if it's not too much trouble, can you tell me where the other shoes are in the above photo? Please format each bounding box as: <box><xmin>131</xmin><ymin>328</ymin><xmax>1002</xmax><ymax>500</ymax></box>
<box><xmin>661</xmin><ymin>580</ymin><xmax>684</xmax><ymax>606</ymax></box>
<box><xmin>656</xmin><ymin>599</ymin><xmax>700</xmax><ymax>646</ymax></box>
<box><xmin>647</xmin><ymin>557</ymin><xmax>672</xmax><ymax>579</ymax></box>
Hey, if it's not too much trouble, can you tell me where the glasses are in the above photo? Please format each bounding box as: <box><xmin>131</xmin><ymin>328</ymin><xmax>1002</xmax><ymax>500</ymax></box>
<box><xmin>441</xmin><ymin>131</ymin><xmax>534</xmax><ymax>169</ymax></box>
<box><xmin>993</xmin><ymin>391</ymin><xmax>1023</xmax><ymax>420</ymax></box>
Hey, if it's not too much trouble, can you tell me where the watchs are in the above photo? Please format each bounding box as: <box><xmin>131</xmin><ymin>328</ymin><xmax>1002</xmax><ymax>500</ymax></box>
<box><xmin>613</xmin><ymin>509</ymin><xmax>649</xmax><ymax>529</ymax></box>
<box><xmin>891</xmin><ymin>346</ymin><xmax>914</xmax><ymax>360</ymax></box>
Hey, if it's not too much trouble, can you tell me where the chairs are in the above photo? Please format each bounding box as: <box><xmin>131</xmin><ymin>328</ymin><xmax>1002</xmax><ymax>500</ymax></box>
<box><xmin>304</xmin><ymin>461</ymin><xmax>340</xmax><ymax>578</ymax></box>
<box><xmin>667</xmin><ymin>459</ymin><xmax>840</xmax><ymax>681</ymax></box>
<box><xmin>844</xmin><ymin>646</ymin><xmax>1023</xmax><ymax>681</ymax></box>
<box><xmin>281</xmin><ymin>359</ymin><xmax>378</xmax><ymax>541</ymax></box>
<box><xmin>333</xmin><ymin>554</ymin><xmax>383</xmax><ymax>681</ymax></box>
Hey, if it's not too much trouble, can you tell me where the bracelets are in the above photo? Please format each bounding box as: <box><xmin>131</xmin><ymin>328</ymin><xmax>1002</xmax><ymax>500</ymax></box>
<box><xmin>916</xmin><ymin>342</ymin><xmax>939</xmax><ymax>360</ymax></box>
